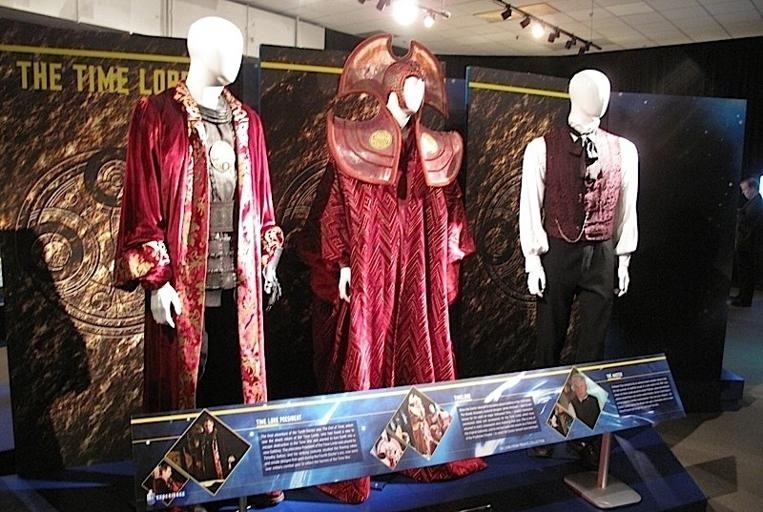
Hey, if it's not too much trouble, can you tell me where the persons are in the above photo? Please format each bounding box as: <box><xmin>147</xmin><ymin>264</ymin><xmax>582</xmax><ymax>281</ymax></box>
<box><xmin>195</xmin><ymin>416</ymin><xmax>237</xmax><ymax>495</ymax></box>
<box><xmin>407</xmin><ymin>392</ymin><xmax>432</xmax><ymax>460</ymax></box>
<box><xmin>426</xmin><ymin>401</ymin><xmax>447</xmax><ymax>443</ymax></box>
<box><xmin>567</xmin><ymin>374</ymin><xmax>602</xmax><ymax>430</ymax></box>
<box><xmin>728</xmin><ymin>178</ymin><xmax>762</xmax><ymax>307</ymax></box>
<box><xmin>550</xmin><ymin>406</ymin><xmax>566</xmax><ymax>437</ymax></box>
<box><xmin>115</xmin><ymin>13</ymin><xmax>288</xmax><ymax>511</ymax></box>
<box><xmin>517</xmin><ymin>72</ymin><xmax>642</xmax><ymax>471</ymax></box>
<box><xmin>322</xmin><ymin>56</ymin><xmax>462</xmax><ymax>486</ymax></box>
<box><xmin>154</xmin><ymin>463</ymin><xmax>178</xmax><ymax>504</ymax></box>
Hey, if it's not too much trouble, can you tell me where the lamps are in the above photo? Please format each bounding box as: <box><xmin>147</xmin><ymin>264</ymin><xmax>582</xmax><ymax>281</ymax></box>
<box><xmin>500</xmin><ymin>3</ymin><xmax>593</xmax><ymax>57</ymax></box>
<box><xmin>357</xmin><ymin>0</ymin><xmax>436</xmax><ymax>28</ymax></box>
